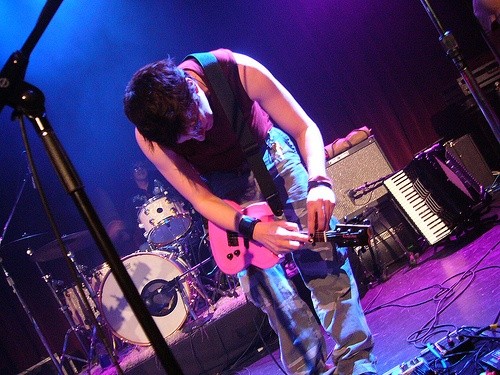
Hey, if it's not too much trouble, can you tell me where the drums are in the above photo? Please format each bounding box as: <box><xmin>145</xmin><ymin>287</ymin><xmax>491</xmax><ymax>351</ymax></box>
<box><xmin>63</xmin><ymin>261</ymin><xmax>110</xmax><ymax>333</ymax></box>
<box><xmin>96</xmin><ymin>251</ymin><xmax>196</xmax><ymax>347</ymax></box>
<box><xmin>137</xmin><ymin>192</ymin><xmax>194</xmax><ymax>247</ymax></box>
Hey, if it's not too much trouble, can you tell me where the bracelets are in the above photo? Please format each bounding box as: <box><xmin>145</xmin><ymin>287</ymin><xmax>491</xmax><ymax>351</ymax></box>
<box><xmin>239</xmin><ymin>216</ymin><xmax>260</xmax><ymax>241</ymax></box>
<box><xmin>307</xmin><ymin>176</ymin><xmax>333</xmax><ymax>192</ymax></box>
<box><xmin>234</xmin><ymin>212</ymin><xmax>244</xmax><ymax>229</ymax></box>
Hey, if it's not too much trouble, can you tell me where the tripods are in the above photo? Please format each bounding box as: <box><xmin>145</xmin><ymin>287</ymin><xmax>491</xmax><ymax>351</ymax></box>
<box><xmin>0</xmin><ymin>240</ymin><xmax>135</xmax><ymax>375</ymax></box>
<box><xmin>167</xmin><ymin>222</ymin><xmax>238</xmax><ymax>309</ymax></box>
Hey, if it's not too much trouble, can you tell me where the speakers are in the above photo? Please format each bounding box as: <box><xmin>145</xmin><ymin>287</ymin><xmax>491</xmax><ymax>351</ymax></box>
<box><xmin>324</xmin><ymin>135</ymin><xmax>395</xmax><ymax>222</ymax></box>
<box><xmin>451</xmin><ymin>133</ymin><xmax>498</xmax><ymax>188</ymax></box>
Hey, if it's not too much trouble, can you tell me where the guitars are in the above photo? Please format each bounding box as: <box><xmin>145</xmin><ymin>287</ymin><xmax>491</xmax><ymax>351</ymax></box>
<box><xmin>207</xmin><ymin>198</ymin><xmax>377</xmax><ymax>277</ymax></box>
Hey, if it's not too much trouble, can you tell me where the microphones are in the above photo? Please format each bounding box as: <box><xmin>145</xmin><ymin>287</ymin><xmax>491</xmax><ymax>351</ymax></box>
<box><xmin>348</xmin><ymin>188</ymin><xmax>358</xmax><ymax>196</ymax></box>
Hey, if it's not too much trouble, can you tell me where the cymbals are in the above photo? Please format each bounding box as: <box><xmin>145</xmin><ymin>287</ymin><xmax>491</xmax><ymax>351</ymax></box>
<box><xmin>35</xmin><ymin>230</ymin><xmax>93</xmax><ymax>262</ymax></box>
<box><xmin>1</xmin><ymin>232</ymin><xmax>47</xmax><ymax>254</ymax></box>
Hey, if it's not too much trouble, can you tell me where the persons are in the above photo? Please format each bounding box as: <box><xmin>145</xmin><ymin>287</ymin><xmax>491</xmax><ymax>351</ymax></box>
<box><xmin>122</xmin><ymin>157</ymin><xmax>169</xmax><ymax>253</ymax></box>
<box><xmin>125</xmin><ymin>49</ymin><xmax>379</xmax><ymax>375</ymax></box>
